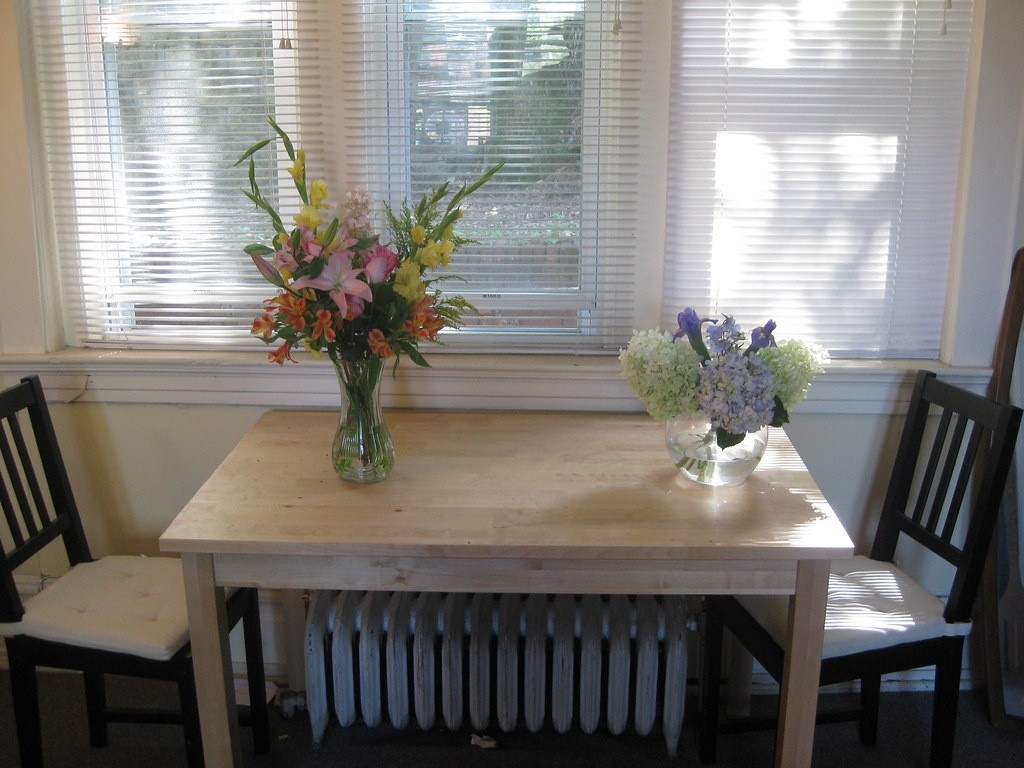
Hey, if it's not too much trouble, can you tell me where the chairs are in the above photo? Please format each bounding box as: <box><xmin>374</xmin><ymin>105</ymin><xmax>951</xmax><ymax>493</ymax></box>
<box><xmin>0</xmin><ymin>374</ymin><xmax>270</xmax><ymax>768</ymax></box>
<box><xmin>696</xmin><ymin>371</ymin><xmax>1024</xmax><ymax>768</ymax></box>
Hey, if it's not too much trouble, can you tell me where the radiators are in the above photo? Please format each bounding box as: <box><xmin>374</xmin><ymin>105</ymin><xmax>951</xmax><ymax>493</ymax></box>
<box><xmin>303</xmin><ymin>591</ymin><xmax>688</xmax><ymax>760</ymax></box>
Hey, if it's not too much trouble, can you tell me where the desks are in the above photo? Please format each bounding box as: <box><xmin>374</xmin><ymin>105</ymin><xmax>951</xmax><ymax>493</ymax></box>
<box><xmin>159</xmin><ymin>409</ymin><xmax>854</xmax><ymax>768</ymax></box>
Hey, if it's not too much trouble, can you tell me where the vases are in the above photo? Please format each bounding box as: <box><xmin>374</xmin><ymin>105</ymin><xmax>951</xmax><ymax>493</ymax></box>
<box><xmin>664</xmin><ymin>413</ymin><xmax>768</xmax><ymax>487</ymax></box>
<box><xmin>330</xmin><ymin>358</ymin><xmax>394</xmax><ymax>485</ymax></box>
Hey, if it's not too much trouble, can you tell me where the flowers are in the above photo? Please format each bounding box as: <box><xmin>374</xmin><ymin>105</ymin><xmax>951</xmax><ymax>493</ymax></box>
<box><xmin>617</xmin><ymin>307</ymin><xmax>831</xmax><ymax>481</ymax></box>
<box><xmin>233</xmin><ymin>118</ymin><xmax>506</xmax><ymax>478</ymax></box>
